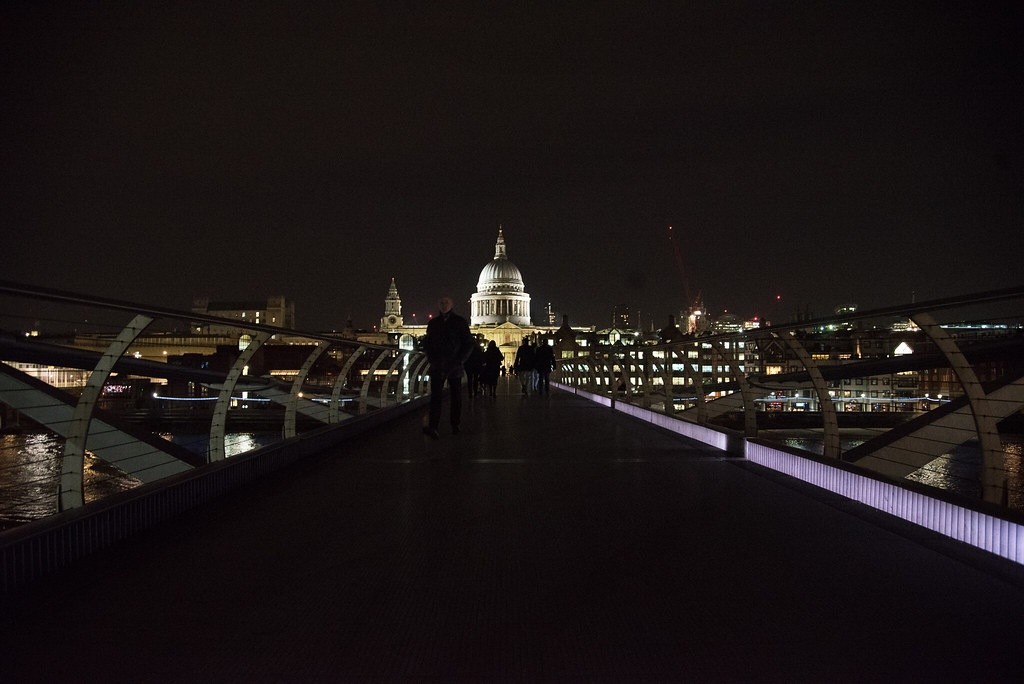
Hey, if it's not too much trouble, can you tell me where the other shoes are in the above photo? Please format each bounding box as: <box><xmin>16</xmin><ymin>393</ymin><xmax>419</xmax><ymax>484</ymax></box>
<box><xmin>522</xmin><ymin>385</ymin><xmax>529</xmax><ymax>399</ymax></box>
<box><xmin>490</xmin><ymin>392</ymin><xmax>496</xmax><ymax>398</ymax></box>
<box><xmin>423</xmin><ymin>426</ymin><xmax>439</xmax><ymax>440</ymax></box>
<box><xmin>452</xmin><ymin>422</ymin><xmax>461</xmax><ymax>434</ymax></box>
<box><xmin>539</xmin><ymin>389</ymin><xmax>550</xmax><ymax>397</ymax></box>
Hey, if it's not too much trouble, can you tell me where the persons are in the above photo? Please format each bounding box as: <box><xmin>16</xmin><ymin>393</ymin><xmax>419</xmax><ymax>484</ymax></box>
<box><xmin>462</xmin><ymin>333</ymin><xmax>555</xmax><ymax>403</ymax></box>
<box><xmin>421</xmin><ymin>293</ymin><xmax>476</xmax><ymax>442</ymax></box>
